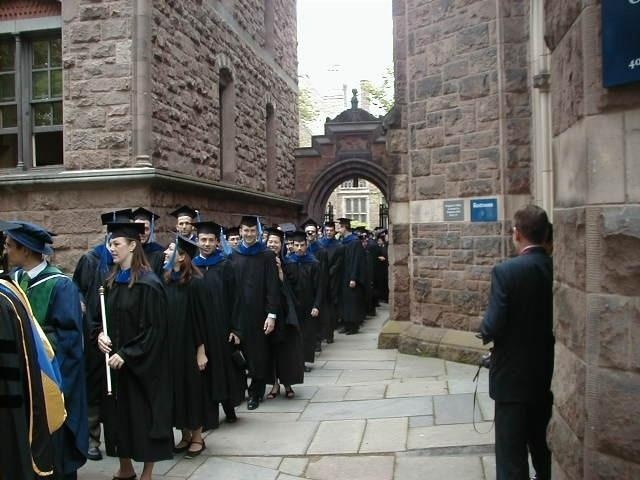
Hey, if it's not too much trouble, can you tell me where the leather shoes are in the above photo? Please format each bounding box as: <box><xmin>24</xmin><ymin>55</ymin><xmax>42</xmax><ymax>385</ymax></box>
<box><xmin>324</xmin><ymin>218</ymin><xmax>388</xmax><ymax>240</ymax></box>
<box><xmin>305</xmin><ymin>326</ymin><xmax>359</xmax><ymax>373</ymax></box>
<box><xmin>184</xmin><ymin>439</ymin><xmax>205</xmax><ymax>459</ymax></box>
<box><xmin>173</xmin><ymin>438</ymin><xmax>191</xmax><ymax>453</ymax></box>
<box><xmin>204</xmin><ymin>393</ymin><xmax>264</xmax><ymax>431</ymax></box>
<box><xmin>105</xmin><ymin>448</ymin><xmax>118</xmax><ymax>456</ymax></box>
<box><xmin>88</xmin><ymin>448</ymin><xmax>103</xmax><ymax>461</ymax></box>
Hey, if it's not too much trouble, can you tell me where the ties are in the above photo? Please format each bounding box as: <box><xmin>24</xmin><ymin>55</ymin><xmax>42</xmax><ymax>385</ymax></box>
<box><xmin>20</xmin><ymin>273</ymin><xmax>30</xmax><ymax>294</ymax></box>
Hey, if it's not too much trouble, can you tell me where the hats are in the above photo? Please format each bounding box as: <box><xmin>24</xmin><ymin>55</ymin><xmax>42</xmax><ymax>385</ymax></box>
<box><xmin>225</xmin><ymin>216</ymin><xmax>318</xmax><ymax>242</ymax></box>
<box><xmin>107</xmin><ymin>222</ymin><xmax>146</xmax><ymax>241</ymax></box>
<box><xmin>169</xmin><ymin>204</ymin><xmax>201</xmax><ymax>219</ymax></box>
<box><xmin>133</xmin><ymin>206</ymin><xmax>160</xmax><ymax>243</ymax></box>
<box><xmin>189</xmin><ymin>221</ymin><xmax>233</xmax><ymax>257</ymax></box>
<box><xmin>100</xmin><ymin>208</ymin><xmax>133</xmax><ymax>226</ymax></box>
<box><xmin>6</xmin><ymin>219</ymin><xmax>54</xmax><ymax>256</ymax></box>
<box><xmin>1</xmin><ymin>220</ymin><xmax>24</xmax><ymax>233</ymax></box>
<box><xmin>163</xmin><ymin>230</ymin><xmax>209</xmax><ymax>272</ymax></box>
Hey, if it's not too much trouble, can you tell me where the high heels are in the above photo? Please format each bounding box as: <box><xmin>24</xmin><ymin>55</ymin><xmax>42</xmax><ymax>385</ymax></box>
<box><xmin>267</xmin><ymin>384</ymin><xmax>280</xmax><ymax>399</ymax></box>
<box><xmin>285</xmin><ymin>387</ymin><xmax>295</xmax><ymax>398</ymax></box>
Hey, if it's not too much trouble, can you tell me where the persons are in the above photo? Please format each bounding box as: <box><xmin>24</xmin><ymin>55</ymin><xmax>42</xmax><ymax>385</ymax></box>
<box><xmin>88</xmin><ymin>221</ymin><xmax>174</xmax><ymax>480</ymax></box>
<box><xmin>0</xmin><ymin>270</ymin><xmax>68</xmax><ymax>480</ymax></box>
<box><xmin>479</xmin><ymin>204</ymin><xmax>555</xmax><ymax>480</ymax></box>
<box><xmin>284</xmin><ymin>217</ymin><xmax>388</xmax><ymax>372</ymax></box>
<box><xmin>2</xmin><ymin>221</ymin><xmax>88</xmax><ymax>480</ymax></box>
<box><xmin>71</xmin><ymin>207</ymin><xmax>134</xmax><ymax>460</ymax></box>
<box><xmin>544</xmin><ymin>223</ymin><xmax>552</xmax><ymax>256</ymax></box>
<box><xmin>133</xmin><ymin>205</ymin><xmax>306</xmax><ymax>457</ymax></box>
